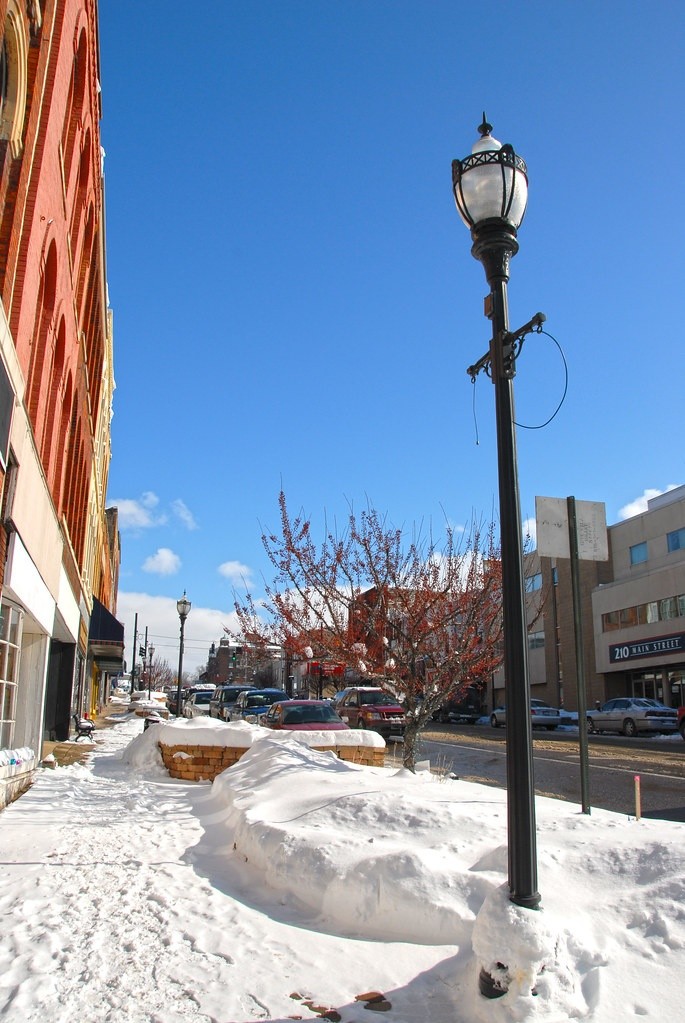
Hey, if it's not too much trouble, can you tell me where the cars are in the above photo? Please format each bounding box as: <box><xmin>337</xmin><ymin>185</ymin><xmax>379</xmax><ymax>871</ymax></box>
<box><xmin>586</xmin><ymin>698</ymin><xmax>678</xmax><ymax>738</ymax></box>
<box><xmin>161</xmin><ymin>685</ymin><xmax>171</xmax><ymax>694</ymax></box>
<box><xmin>184</xmin><ymin>693</ymin><xmax>213</xmax><ymax>718</ymax></box>
<box><xmin>114</xmin><ymin>688</ymin><xmax>127</xmax><ymax>698</ymax></box>
<box><xmin>225</xmin><ymin>690</ymin><xmax>291</xmax><ymax>723</ymax></box>
<box><xmin>490</xmin><ymin>699</ymin><xmax>561</xmax><ymax>731</ymax></box>
<box><xmin>677</xmin><ymin>708</ymin><xmax>685</xmax><ymax>740</ymax></box>
<box><xmin>260</xmin><ymin>700</ymin><xmax>351</xmax><ymax>731</ymax></box>
<box><xmin>330</xmin><ymin>692</ymin><xmax>343</xmax><ymax>710</ymax></box>
<box><xmin>165</xmin><ymin>690</ymin><xmax>185</xmax><ymax>715</ymax></box>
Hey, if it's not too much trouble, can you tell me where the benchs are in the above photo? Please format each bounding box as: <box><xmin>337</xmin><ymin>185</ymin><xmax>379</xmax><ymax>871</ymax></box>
<box><xmin>73</xmin><ymin>713</ymin><xmax>95</xmax><ymax>742</ymax></box>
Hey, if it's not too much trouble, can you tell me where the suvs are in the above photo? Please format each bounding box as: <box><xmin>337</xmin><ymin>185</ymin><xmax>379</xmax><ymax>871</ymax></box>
<box><xmin>336</xmin><ymin>687</ymin><xmax>417</xmax><ymax>738</ymax></box>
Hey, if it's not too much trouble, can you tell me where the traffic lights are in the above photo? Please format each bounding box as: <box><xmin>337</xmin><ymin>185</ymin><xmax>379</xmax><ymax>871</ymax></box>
<box><xmin>232</xmin><ymin>653</ymin><xmax>236</xmax><ymax>660</ymax></box>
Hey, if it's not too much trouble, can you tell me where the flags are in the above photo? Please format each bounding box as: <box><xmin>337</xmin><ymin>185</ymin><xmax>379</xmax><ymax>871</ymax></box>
<box><xmin>139</xmin><ymin>647</ymin><xmax>145</xmax><ymax>657</ymax></box>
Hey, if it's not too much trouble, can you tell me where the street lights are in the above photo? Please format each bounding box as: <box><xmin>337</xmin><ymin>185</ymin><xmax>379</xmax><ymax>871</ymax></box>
<box><xmin>176</xmin><ymin>590</ymin><xmax>192</xmax><ymax>717</ymax></box>
<box><xmin>147</xmin><ymin>643</ymin><xmax>156</xmax><ymax>700</ymax></box>
<box><xmin>451</xmin><ymin>113</ymin><xmax>547</xmax><ymax>912</ymax></box>
<box><xmin>142</xmin><ymin>658</ymin><xmax>147</xmax><ymax>671</ymax></box>
<box><xmin>289</xmin><ymin>676</ymin><xmax>294</xmax><ymax>697</ymax></box>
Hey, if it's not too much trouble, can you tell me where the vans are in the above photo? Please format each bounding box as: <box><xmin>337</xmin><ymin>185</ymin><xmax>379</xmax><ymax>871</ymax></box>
<box><xmin>182</xmin><ymin>685</ymin><xmax>216</xmax><ymax>713</ymax></box>
<box><xmin>208</xmin><ymin>686</ymin><xmax>258</xmax><ymax>722</ymax></box>
<box><xmin>431</xmin><ymin>686</ymin><xmax>481</xmax><ymax>724</ymax></box>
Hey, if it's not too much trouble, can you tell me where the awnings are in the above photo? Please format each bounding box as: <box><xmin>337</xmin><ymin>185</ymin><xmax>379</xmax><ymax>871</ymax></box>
<box><xmin>88</xmin><ymin>594</ymin><xmax>124</xmax><ymax>677</ymax></box>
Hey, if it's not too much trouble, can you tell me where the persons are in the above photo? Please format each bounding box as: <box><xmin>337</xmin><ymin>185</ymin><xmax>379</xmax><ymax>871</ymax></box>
<box><xmin>677</xmin><ymin>706</ymin><xmax>685</xmax><ymax>742</ymax></box>
